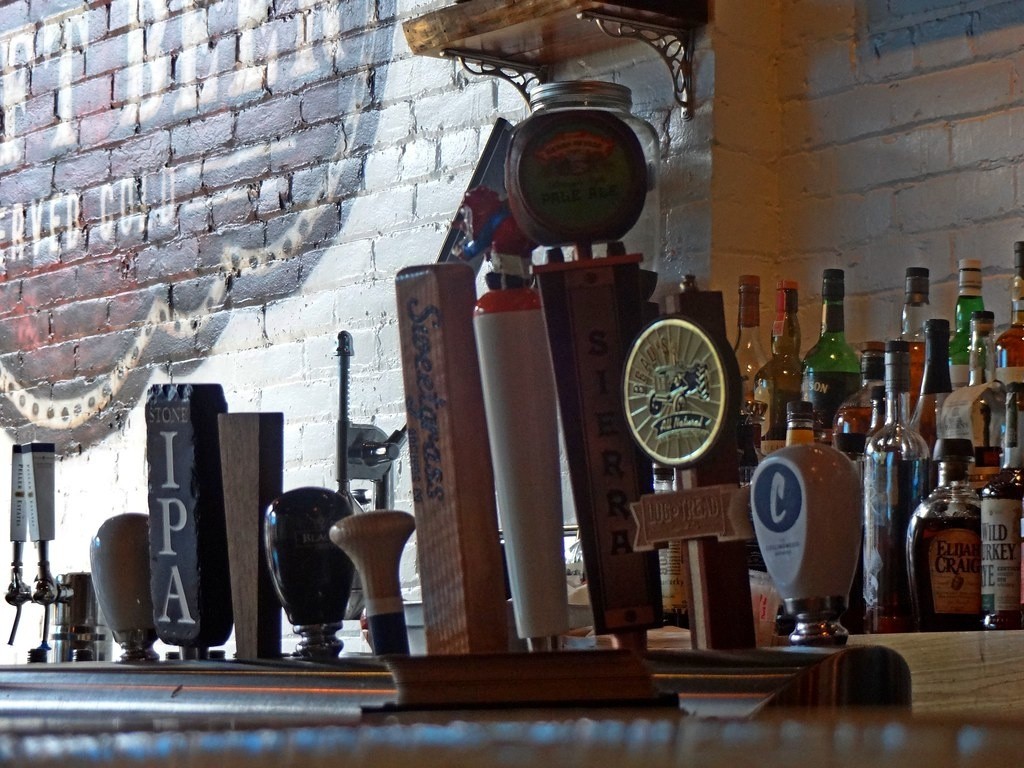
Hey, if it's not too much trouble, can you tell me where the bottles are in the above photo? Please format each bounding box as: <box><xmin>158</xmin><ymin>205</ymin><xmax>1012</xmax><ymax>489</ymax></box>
<box><xmin>732</xmin><ymin>240</ymin><xmax>1024</xmax><ymax>648</ymax></box>
